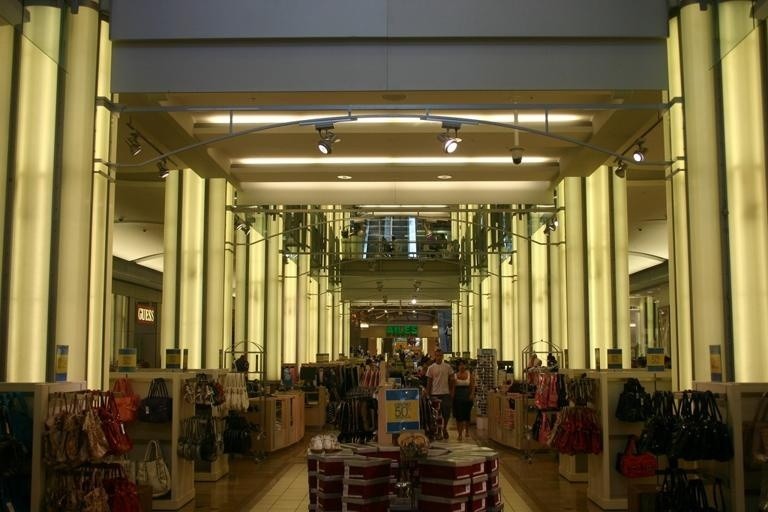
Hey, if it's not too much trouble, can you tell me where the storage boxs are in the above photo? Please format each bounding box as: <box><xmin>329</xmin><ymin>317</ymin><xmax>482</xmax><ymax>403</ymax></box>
<box><xmin>340</xmin><ymin>477</ymin><xmax>391</xmax><ymax>498</ymax></box>
<box><xmin>309</xmin><ymin>489</ymin><xmax>317</xmax><ymax>505</ymax></box>
<box><xmin>487</xmin><ymin>490</ymin><xmax>500</xmax><ymax>507</ymax></box>
<box><xmin>308</xmin><ymin>504</ymin><xmax>317</xmax><ymax>511</ymax></box>
<box><xmin>340</xmin><ymin>497</ymin><xmax>396</xmax><ymax>511</ymax></box>
<box><xmin>317</xmin><ymin>455</ymin><xmax>367</xmax><ymax>475</ymax></box>
<box><xmin>315</xmin><ymin>493</ymin><xmax>342</xmax><ymax>507</ymax></box>
<box><xmin>421</xmin><ymin>478</ymin><xmax>472</xmax><ymax>496</ymax></box>
<box><xmin>446</xmin><ymin>450</ymin><xmax>499</xmax><ymax>473</ymax></box>
<box><xmin>440</xmin><ymin>455</ymin><xmax>486</xmax><ymax>475</ymax></box>
<box><xmin>343</xmin><ymin>457</ymin><xmax>393</xmax><ymax>476</ymax></box>
<box><xmin>472</xmin><ymin>474</ymin><xmax>487</xmax><ymax>492</ymax></box>
<box><xmin>470</xmin><ymin>492</ymin><xmax>486</xmax><ymax>510</ymax></box>
<box><xmin>416</xmin><ymin>497</ymin><xmax>468</xmax><ymax>511</ymax></box>
<box><xmin>306</xmin><ymin>447</ymin><xmax>355</xmax><ymax>472</ymax></box>
<box><xmin>308</xmin><ymin>473</ymin><xmax>316</xmax><ymax>488</ymax></box>
<box><xmin>372</xmin><ymin>442</ymin><xmax>399</xmax><ymax>457</ymax></box>
<box><xmin>417</xmin><ymin>458</ymin><xmax>473</xmax><ymax>478</ymax></box>
<box><xmin>316</xmin><ymin>476</ymin><xmax>346</xmax><ymax>492</ymax></box>
<box><xmin>342</xmin><ymin>441</ymin><xmax>377</xmax><ymax>457</ymax></box>
<box><xmin>487</xmin><ymin>473</ymin><xmax>499</xmax><ymax>487</ymax></box>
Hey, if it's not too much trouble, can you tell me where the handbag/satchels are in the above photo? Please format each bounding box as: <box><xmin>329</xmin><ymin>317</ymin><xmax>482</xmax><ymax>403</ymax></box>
<box><xmin>138</xmin><ymin>378</ymin><xmax>173</xmax><ymax>424</ymax></box>
<box><xmin>185</xmin><ymin>373</ymin><xmax>226</xmax><ymax>407</ymax></box>
<box><xmin>615</xmin><ymin>435</ymin><xmax>657</xmax><ymax>478</ymax></box>
<box><xmin>633</xmin><ymin>391</ymin><xmax>735</xmax><ymax>462</ymax></box>
<box><xmin>137</xmin><ymin>440</ymin><xmax>171</xmax><ymax>497</ymax></box>
<box><xmin>111</xmin><ymin>377</ymin><xmax>140</xmax><ymax>423</ymax></box>
<box><xmin>0</xmin><ymin>391</ymin><xmax>33</xmax><ymax>504</ymax></box>
<box><xmin>615</xmin><ymin>378</ymin><xmax>654</xmax><ymax>423</ymax></box>
<box><xmin>41</xmin><ymin>389</ymin><xmax>133</xmax><ymax>463</ymax></box>
<box><xmin>119</xmin><ymin>454</ymin><xmax>135</xmax><ymax>482</ymax></box>
<box><xmin>223</xmin><ymin>416</ymin><xmax>259</xmax><ymax>454</ymax></box>
<box><xmin>177</xmin><ymin>415</ymin><xmax>219</xmax><ymax>462</ymax></box>
<box><xmin>638</xmin><ymin>467</ymin><xmax>728</xmax><ymax>512</ymax></box>
<box><xmin>217</xmin><ymin>372</ymin><xmax>249</xmax><ymax>412</ymax></box>
<box><xmin>744</xmin><ymin>391</ymin><xmax>768</xmax><ymax>472</ymax></box>
<box><xmin>41</xmin><ymin>466</ymin><xmax>145</xmax><ymax>512</ymax></box>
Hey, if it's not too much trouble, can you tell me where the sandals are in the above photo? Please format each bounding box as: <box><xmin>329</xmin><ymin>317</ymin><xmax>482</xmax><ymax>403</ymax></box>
<box><xmin>324</xmin><ymin>434</ymin><xmax>342</xmax><ymax>453</ymax></box>
<box><xmin>414</xmin><ymin>433</ymin><xmax>429</xmax><ymax>458</ymax></box>
<box><xmin>398</xmin><ymin>432</ymin><xmax>415</xmax><ymax>457</ymax></box>
<box><xmin>309</xmin><ymin>435</ymin><xmax>322</xmax><ymax>454</ymax></box>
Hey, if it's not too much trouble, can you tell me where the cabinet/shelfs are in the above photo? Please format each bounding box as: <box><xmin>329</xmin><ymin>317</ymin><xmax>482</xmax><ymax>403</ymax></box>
<box><xmin>192</xmin><ymin>367</ymin><xmax>233</xmax><ymax>482</ymax></box>
<box><xmin>295</xmin><ymin>358</ymin><xmax>347</xmax><ymax>426</ymax></box>
<box><xmin>0</xmin><ymin>380</ymin><xmax>100</xmax><ymax>512</ymax></box>
<box><xmin>558</xmin><ymin>365</ymin><xmax>597</xmax><ymax>490</ymax></box>
<box><xmin>583</xmin><ymin>367</ymin><xmax>675</xmax><ymax>507</ymax></box>
<box><xmin>249</xmin><ymin>383</ymin><xmax>311</xmax><ymax>459</ymax></box>
<box><xmin>108</xmin><ymin>369</ymin><xmax>199</xmax><ymax>509</ymax></box>
<box><xmin>483</xmin><ymin>388</ymin><xmax>539</xmax><ymax>455</ymax></box>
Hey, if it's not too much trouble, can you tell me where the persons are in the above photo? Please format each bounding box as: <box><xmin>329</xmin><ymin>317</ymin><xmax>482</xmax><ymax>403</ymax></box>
<box><xmin>417</xmin><ymin>356</ymin><xmax>431</xmax><ymax>377</ymax></box>
<box><xmin>450</xmin><ymin>358</ymin><xmax>475</xmax><ymax>441</ymax></box>
<box><xmin>424</xmin><ymin>349</ymin><xmax>456</xmax><ymax>440</ymax></box>
<box><xmin>374</xmin><ymin>359</ymin><xmax>380</xmax><ymax>367</ymax></box>
<box><xmin>360</xmin><ymin>350</ymin><xmax>384</xmax><ymax>371</ymax></box>
